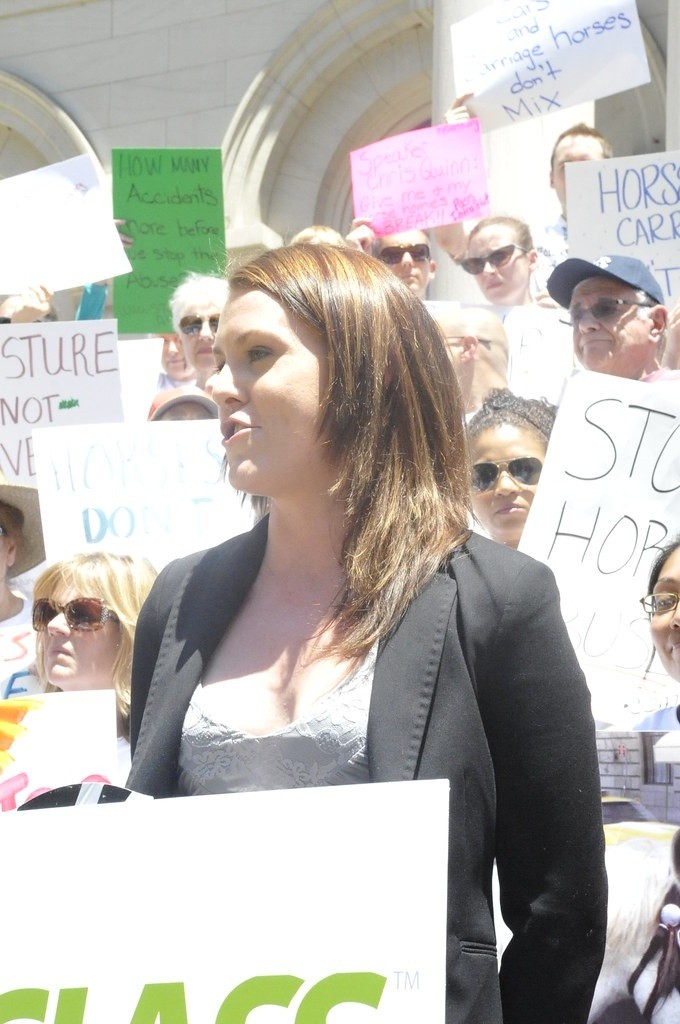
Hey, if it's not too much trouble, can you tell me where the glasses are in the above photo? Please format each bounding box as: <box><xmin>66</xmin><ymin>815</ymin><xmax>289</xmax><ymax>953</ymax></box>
<box><xmin>461</xmin><ymin>244</ymin><xmax>530</xmax><ymax>275</ymax></box>
<box><xmin>640</xmin><ymin>591</ymin><xmax>680</xmax><ymax>615</ymax></box>
<box><xmin>468</xmin><ymin>454</ymin><xmax>543</xmax><ymax>491</ymax></box>
<box><xmin>0</xmin><ymin>524</ymin><xmax>15</xmax><ymax>539</ymax></box>
<box><xmin>560</xmin><ymin>296</ymin><xmax>658</xmax><ymax>329</ymax></box>
<box><xmin>177</xmin><ymin>313</ymin><xmax>221</xmax><ymax>335</ymax></box>
<box><xmin>32</xmin><ymin>596</ymin><xmax>119</xmax><ymax>634</ymax></box>
<box><xmin>375</xmin><ymin>242</ymin><xmax>433</xmax><ymax>264</ymax></box>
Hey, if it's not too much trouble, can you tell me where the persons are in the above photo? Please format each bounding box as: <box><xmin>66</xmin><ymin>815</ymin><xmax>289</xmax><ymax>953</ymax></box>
<box><xmin>425</xmin><ymin>302</ymin><xmax>506</xmax><ymax>408</ymax></box>
<box><xmin>1</xmin><ymin>482</ymin><xmax>53</xmax><ymax>696</ymax></box>
<box><xmin>460</xmin><ymin>212</ymin><xmax>535</xmax><ymax>307</ymax></box>
<box><xmin>457</xmin><ymin>373</ymin><xmax>560</xmax><ymax>547</ymax></box>
<box><xmin>122</xmin><ymin>244</ymin><xmax>608</xmax><ymax>1024</ymax></box>
<box><xmin>267</xmin><ymin>217</ymin><xmax>448</xmax><ymax>296</ymax></box>
<box><xmin>149</xmin><ymin>267</ymin><xmax>232</xmax><ymax>417</ymax></box>
<box><xmin>3</xmin><ymin>267</ymin><xmax>58</xmax><ymax>333</ymax></box>
<box><xmin>550</xmin><ymin>121</ymin><xmax>616</xmax><ymax>238</ymax></box>
<box><xmin>31</xmin><ymin>554</ymin><xmax>159</xmax><ymax>738</ymax></box>
<box><xmin>642</xmin><ymin>536</ymin><xmax>680</xmax><ymax>736</ymax></box>
<box><xmin>553</xmin><ymin>244</ymin><xmax>673</xmax><ymax>381</ymax></box>
<box><xmin>441</xmin><ymin>93</ymin><xmax>474</xmax><ymax>130</ymax></box>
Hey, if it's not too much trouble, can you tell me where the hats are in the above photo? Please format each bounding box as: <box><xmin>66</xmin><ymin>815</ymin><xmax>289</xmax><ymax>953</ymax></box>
<box><xmin>546</xmin><ymin>253</ymin><xmax>665</xmax><ymax>312</ymax></box>
<box><xmin>146</xmin><ymin>386</ymin><xmax>219</xmax><ymax>421</ymax></box>
<box><xmin>0</xmin><ymin>470</ymin><xmax>48</xmax><ymax>579</ymax></box>
